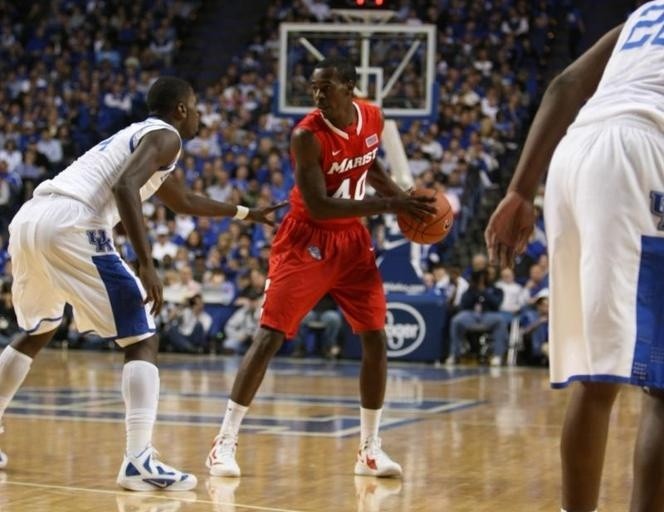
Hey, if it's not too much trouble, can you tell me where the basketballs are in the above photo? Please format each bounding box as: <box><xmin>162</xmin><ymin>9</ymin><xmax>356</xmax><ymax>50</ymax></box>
<box><xmin>399</xmin><ymin>188</ymin><xmax>454</xmax><ymax>244</ymax></box>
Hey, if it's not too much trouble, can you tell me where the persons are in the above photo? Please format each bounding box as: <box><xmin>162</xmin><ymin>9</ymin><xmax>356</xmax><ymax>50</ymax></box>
<box><xmin>0</xmin><ymin>1</ymin><xmax>602</xmax><ymax>370</ymax></box>
<box><xmin>204</xmin><ymin>56</ymin><xmax>440</xmax><ymax>479</ymax></box>
<box><xmin>0</xmin><ymin>73</ymin><xmax>292</xmax><ymax>495</ymax></box>
<box><xmin>484</xmin><ymin>3</ymin><xmax>663</xmax><ymax>512</ymax></box>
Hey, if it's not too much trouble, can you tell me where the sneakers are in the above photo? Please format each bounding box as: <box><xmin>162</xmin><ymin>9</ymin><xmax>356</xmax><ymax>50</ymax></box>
<box><xmin>204</xmin><ymin>434</ymin><xmax>243</xmax><ymax>478</ymax></box>
<box><xmin>116</xmin><ymin>445</ymin><xmax>200</xmax><ymax>494</ymax></box>
<box><xmin>352</xmin><ymin>445</ymin><xmax>401</xmax><ymax>479</ymax></box>
